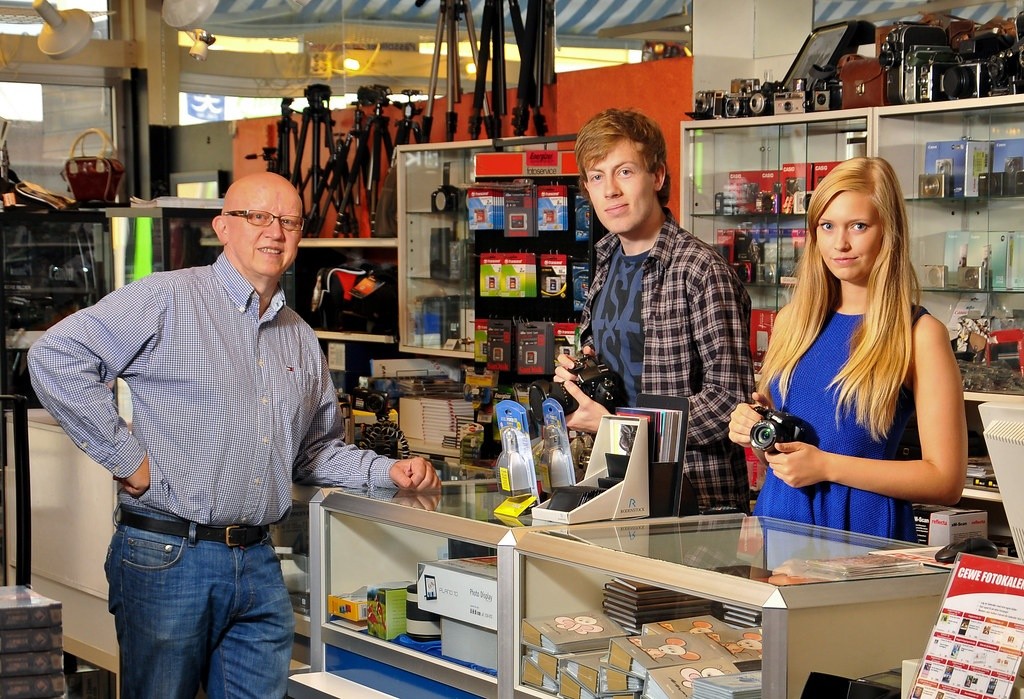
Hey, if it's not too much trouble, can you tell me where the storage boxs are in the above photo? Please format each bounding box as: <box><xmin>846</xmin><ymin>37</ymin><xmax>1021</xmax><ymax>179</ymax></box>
<box><xmin>0</xmin><ymin>580</ymin><xmax>65</xmax><ymax>699</ymax></box>
<box><xmin>326</xmin><ymin>594</ymin><xmax>368</xmax><ymax>622</ymax></box>
<box><xmin>727</xmin><ymin>169</ymin><xmax>781</xmax><ymax>212</ymax></box>
<box><xmin>811</xmin><ymin>161</ymin><xmax>837</xmax><ymax>197</ymax></box>
<box><xmin>780</xmin><ymin>161</ymin><xmax>813</xmax><ymax>210</ymax></box>
<box><xmin>749</xmin><ymin>306</ymin><xmax>778</xmax><ymax>361</ymax></box>
<box><xmin>909</xmin><ymin>502</ymin><xmax>989</xmax><ymax>547</ymax></box>
<box><xmin>365</xmin><ymin>584</ymin><xmax>408</xmax><ymax>640</ymax></box>
<box><xmin>416</xmin><ymin>558</ymin><xmax>498</xmax><ymax>634</ymax></box>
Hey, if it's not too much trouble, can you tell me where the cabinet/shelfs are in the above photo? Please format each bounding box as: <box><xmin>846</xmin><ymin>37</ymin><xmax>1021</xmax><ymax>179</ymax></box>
<box><xmin>680</xmin><ymin>107</ymin><xmax>872</xmax><ymax>362</ymax></box>
<box><xmin>872</xmin><ymin>95</ymin><xmax>1024</xmax><ymax>509</ymax></box>
<box><xmin>395</xmin><ymin>138</ymin><xmax>544</xmax><ymax>360</ymax></box>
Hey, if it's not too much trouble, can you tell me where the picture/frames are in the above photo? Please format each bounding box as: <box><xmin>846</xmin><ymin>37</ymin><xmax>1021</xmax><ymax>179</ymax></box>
<box><xmin>780</xmin><ymin>19</ymin><xmax>855</xmax><ymax>92</ymax></box>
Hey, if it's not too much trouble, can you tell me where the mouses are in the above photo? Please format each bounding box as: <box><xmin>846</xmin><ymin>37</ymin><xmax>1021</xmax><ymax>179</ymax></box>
<box><xmin>934</xmin><ymin>538</ymin><xmax>998</xmax><ymax>563</ymax></box>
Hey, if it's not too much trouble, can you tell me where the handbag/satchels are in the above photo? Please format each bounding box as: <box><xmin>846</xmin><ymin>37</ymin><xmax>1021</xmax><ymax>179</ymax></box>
<box><xmin>310</xmin><ymin>260</ymin><xmax>402</xmax><ymax>338</ymax></box>
<box><xmin>65</xmin><ymin>127</ymin><xmax>127</xmax><ymax>203</ymax></box>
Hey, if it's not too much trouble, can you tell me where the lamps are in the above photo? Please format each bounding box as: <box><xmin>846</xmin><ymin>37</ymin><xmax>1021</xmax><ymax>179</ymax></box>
<box><xmin>31</xmin><ymin>0</ymin><xmax>94</xmax><ymax>61</ymax></box>
<box><xmin>160</xmin><ymin>0</ymin><xmax>220</xmax><ymax>61</ymax></box>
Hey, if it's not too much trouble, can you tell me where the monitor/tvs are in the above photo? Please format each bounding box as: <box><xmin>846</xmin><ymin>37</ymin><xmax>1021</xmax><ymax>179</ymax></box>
<box><xmin>978</xmin><ymin>402</ymin><xmax>1024</xmax><ymax>563</ymax></box>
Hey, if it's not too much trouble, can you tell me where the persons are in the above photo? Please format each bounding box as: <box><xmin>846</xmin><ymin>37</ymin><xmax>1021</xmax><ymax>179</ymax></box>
<box><xmin>27</xmin><ymin>172</ymin><xmax>442</xmax><ymax>699</ymax></box>
<box><xmin>726</xmin><ymin>158</ymin><xmax>969</xmax><ymax>545</ymax></box>
<box><xmin>553</xmin><ymin>109</ymin><xmax>758</xmax><ymax>521</ymax></box>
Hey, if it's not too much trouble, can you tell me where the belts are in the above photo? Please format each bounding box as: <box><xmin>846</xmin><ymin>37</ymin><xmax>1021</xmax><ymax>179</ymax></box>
<box><xmin>118</xmin><ymin>508</ymin><xmax>272</xmax><ymax>548</ymax></box>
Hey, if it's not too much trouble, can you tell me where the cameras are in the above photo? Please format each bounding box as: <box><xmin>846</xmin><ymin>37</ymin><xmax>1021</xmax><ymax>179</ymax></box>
<box><xmin>936</xmin><ymin>158</ymin><xmax>954</xmax><ymax>175</ymax></box>
<box><xmin>352</xmin><ymin>386</ymin><xmax>389</xmax><ymax>413</ymax></box>
<box><xmin>917</xmin><ymin>172</ymin><xmax>954</xmax><ymax>198</ymax></box>
<box><xmin>923</xmin><ymin>265</ymin><xmax>949</xmax><ymax>289</ymax></box>
<box><xmin>978</xmin><ymin>172</ymin><xmax>1010</xmax><ymax>196</ymax></box>
<box><xmin>729</xmin><ymin>261</ymin><xmax>776</xmax><ymax>285</ymax></box>
<box><xmin>956</xmin><ymin>266</ymin><xmax>985</xmax><ymax>289</ymax></box>
<box><xmin>529</xmin><ymin>354</ymin><xmax>621</xmax><ymax>423</ymax></box>
<box><xmin>751</xmin><ymin>191</ymin><xmax>815</xmax><ymax>215</ymax></box>
<box><xmin>684</xmin><ymin>9</ymin><xmax>1024</xmax><ymax>120</ymax></box>
<box><xmin>750</xmin><ymin>407</ymin><xmax>816</xmax><ymax>453</ymax></box>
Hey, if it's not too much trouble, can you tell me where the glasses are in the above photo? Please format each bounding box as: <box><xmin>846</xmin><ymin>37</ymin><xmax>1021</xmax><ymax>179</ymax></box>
<box><xmin>225</xmin><ymin>208</ymin><xmax>305</xmax><ymax>234</ymax></box>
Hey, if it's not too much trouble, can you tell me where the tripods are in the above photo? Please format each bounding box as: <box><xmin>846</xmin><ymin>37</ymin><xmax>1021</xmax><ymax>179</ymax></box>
<box><xmin>245</xmin><ymin>1</ymin><xmax>557</xmax><ymax>236</ymax></box>
